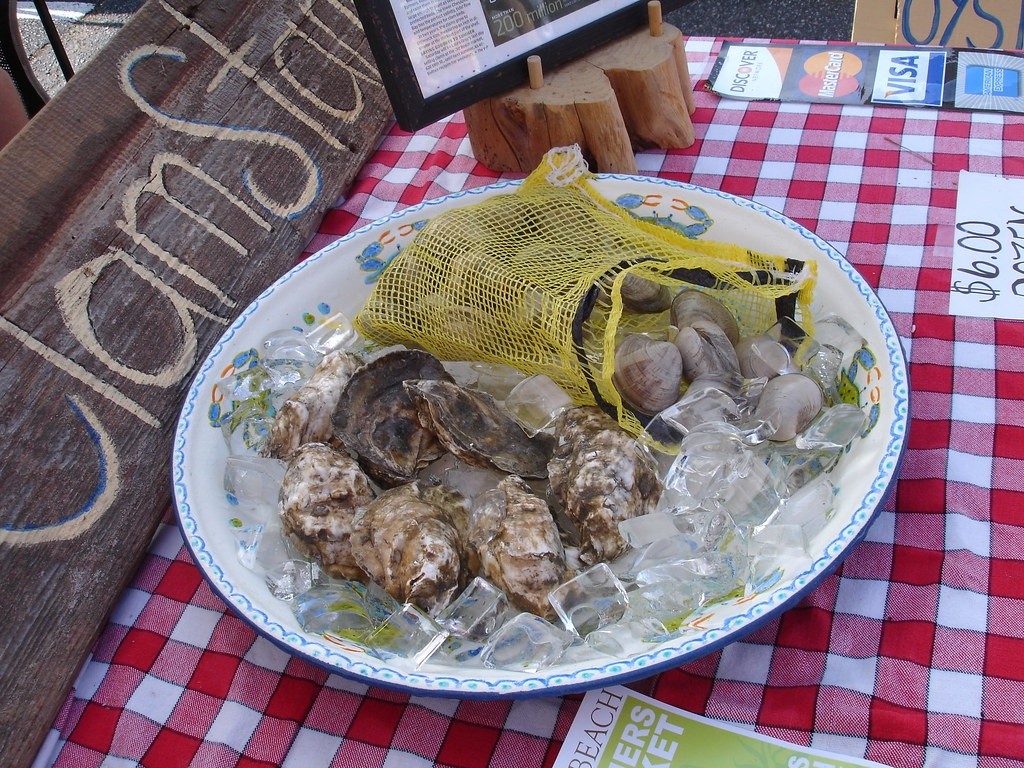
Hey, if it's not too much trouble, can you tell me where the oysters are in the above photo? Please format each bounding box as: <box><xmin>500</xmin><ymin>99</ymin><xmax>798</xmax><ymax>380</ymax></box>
<box><xmin>259</xmin><ymin>349</ymin><xmax>662</xmax><ymax>624</ymax></box>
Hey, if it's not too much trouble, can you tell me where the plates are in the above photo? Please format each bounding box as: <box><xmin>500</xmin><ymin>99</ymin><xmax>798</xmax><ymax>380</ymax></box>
<box><xmin>168</xmin><ymin>175</ymin><xmax>912</xmax><ymax>702</ymax></box>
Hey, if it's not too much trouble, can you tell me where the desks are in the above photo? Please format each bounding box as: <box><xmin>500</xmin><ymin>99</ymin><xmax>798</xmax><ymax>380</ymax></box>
<box><xmin>0</xmin><ymin>36</ymin><xmax>1024</xmax><ymax>768</ymax></box>
